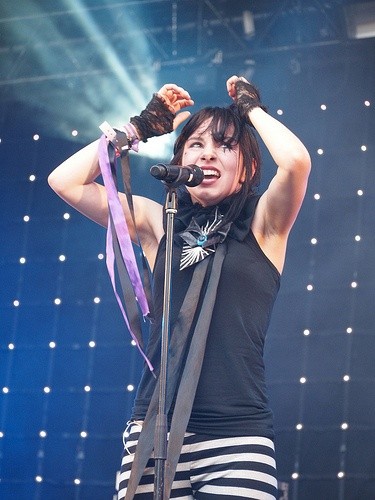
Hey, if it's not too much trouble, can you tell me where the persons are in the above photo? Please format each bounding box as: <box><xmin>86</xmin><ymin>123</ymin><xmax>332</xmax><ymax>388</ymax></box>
<box><xmin>45</xmin><ymin>75</ymin><xmax>312</xmax><ymax>500</ymax></box>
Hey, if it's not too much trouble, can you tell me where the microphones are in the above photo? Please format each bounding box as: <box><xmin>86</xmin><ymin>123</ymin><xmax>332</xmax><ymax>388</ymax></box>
<box><xmin>149</xmin><ymin>163</ymin><xmax>205</xmax><ymax>186</ymax></box>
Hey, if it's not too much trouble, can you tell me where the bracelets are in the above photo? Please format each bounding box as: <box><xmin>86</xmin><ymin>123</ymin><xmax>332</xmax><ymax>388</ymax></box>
<box><xmin>98</xmin><ymin>121</ymin><xmax>131</xmax><ymax>154</ymax></box>
